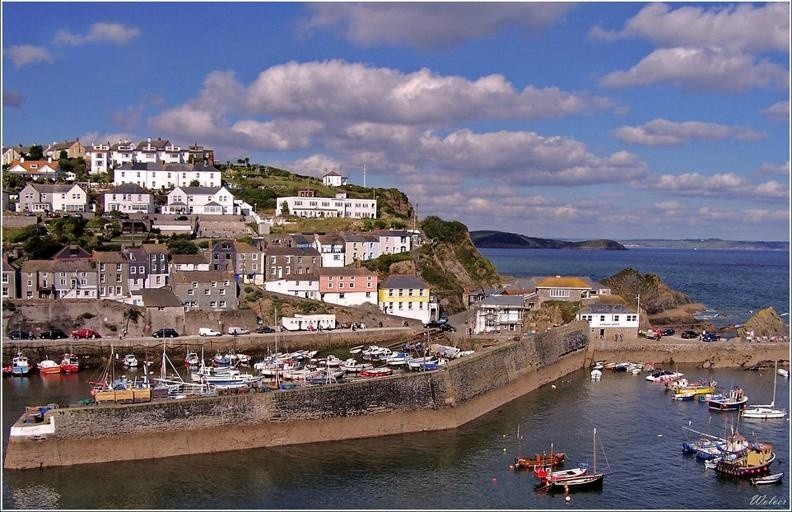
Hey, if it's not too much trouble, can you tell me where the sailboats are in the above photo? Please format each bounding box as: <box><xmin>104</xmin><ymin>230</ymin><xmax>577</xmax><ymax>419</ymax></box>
<box><xmin>85</xmin><ymin>340</ymin><xmax>266</xmax><ymax>402</ymax></box>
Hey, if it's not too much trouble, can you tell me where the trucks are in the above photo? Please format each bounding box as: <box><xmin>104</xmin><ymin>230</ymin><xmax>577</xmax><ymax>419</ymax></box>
<box><xmin>229</xmin><ymin>326</ymin><xmax>250</xmax><ymax>334</ymax></box>
<box><xmin>199</xmin><ymin>327</ymin><xmax>222</xmax><ymax>336</ymax></box>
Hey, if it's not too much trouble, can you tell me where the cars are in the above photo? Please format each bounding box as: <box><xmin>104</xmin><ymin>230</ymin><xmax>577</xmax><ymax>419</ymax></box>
<box><xmin>10</xmin><ymin>327</ymin><xmax>101</xmax><ymax>339</ymax></box>
<box><xmin>661</xmin><ymin>328</ymin><xmax>675</xmax><ymax>336</ymax></box>
<box><xmin>681</xmin><ymin>330</ymin><xmax>698</xmax><ymax>339</ymax></box>
<box><xmin>704</xmin><ymin>333</ymin><xmax>720</xmax><ymax>341</ymax></box>
<box><xmin>153</xmin><ymin>328</ymin><xmax>179</xmax><ymax>337</ymax></box>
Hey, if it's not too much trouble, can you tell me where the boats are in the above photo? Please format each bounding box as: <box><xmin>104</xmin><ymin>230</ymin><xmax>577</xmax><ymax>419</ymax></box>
<box><xmin>740</xmin><ymin>361</ymin><xmax>789</xmax><ymax>418</ymax></box>
<box><xmin>682</xmin><ymin>414</ymin><xmax>784</xmax><ymax>486</ymax></box>
<box><xmin>10</xmin><ymin>342</ymin><xmax>80</xmax><ymax>376</ymax></box>
<box><xmin>519</xmin><ymin>427</ymin><xmax>613</xmax><ymax>489</ymax></box>
<box><xmin>590</xmin><ymin>360</ymin><xmax>645</xmax><ymax>380</ymax></box>
<box><xmin>647</xmin><ymin>370</ymin><xmax>748</xmax><ymax>410</ymax></box>
<box><xmin>254</xmin><ymin>307</ymin><xmax>476</xmax><ymax>387</ymax></box>
